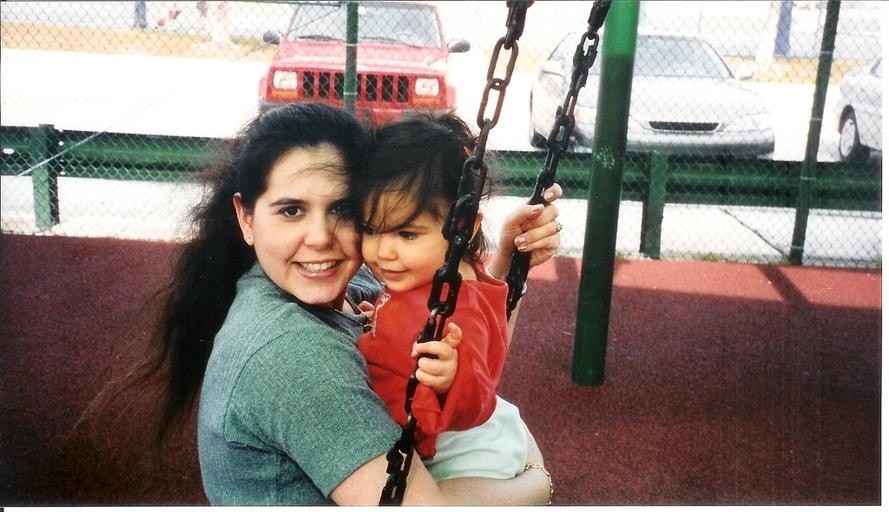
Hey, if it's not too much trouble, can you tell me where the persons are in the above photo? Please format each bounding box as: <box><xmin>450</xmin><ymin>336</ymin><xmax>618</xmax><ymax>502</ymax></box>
<box><xmin>337</xmin><ymin>111</ymin><xmax>544</xmax><ymax>483</ymax></box>
<box><xmin>77</xmin><ymin>103</ymin><xmax>563</xmax><ymax>506</ymax></box>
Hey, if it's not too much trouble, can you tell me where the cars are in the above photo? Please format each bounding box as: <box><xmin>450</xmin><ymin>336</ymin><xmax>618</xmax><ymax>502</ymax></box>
<box><xmin>258</xmin><ymin>0</ymin><xmax>471</xmax><ymax>156</ymax></box>
<box><xmin>834</xmin><ymin>52</ymin><xmax>883</xmax><ymax>162</ymax></box>
<box><xmin>527</xmin><ymin>30</ymin><xmax>777</xmax><ymax>160</ymax></box>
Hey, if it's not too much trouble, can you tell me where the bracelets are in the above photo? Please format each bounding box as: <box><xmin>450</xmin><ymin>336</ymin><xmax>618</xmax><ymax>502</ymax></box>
<box><xmin>484</xmin><ymin>264</ymin><xmax>527</xmax><ymax>296</ymax></box>
<box><xmin>525</xmin><ymin>464</ymin><xmax>554</xmax><ymax>505</ymax></box>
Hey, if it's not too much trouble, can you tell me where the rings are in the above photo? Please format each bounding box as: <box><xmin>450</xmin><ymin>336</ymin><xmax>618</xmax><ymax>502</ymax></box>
<box><xmin>552</xmin><ymin>219</ymin><xmax>562</xmax><ymax>234</ymax></box>
